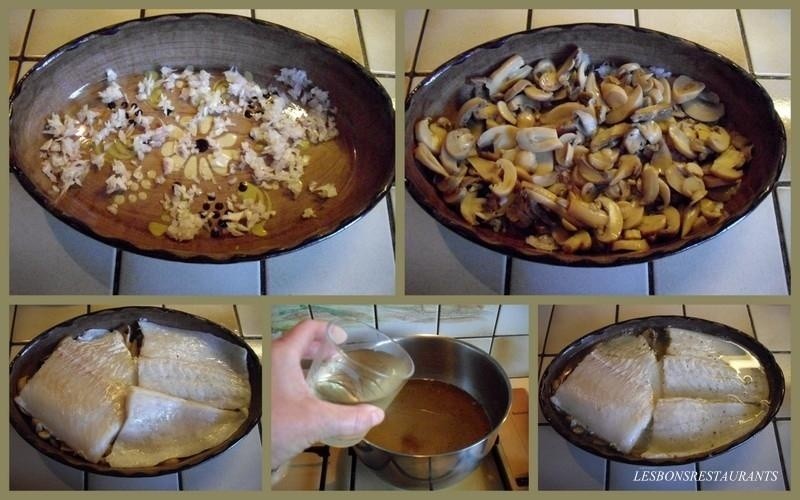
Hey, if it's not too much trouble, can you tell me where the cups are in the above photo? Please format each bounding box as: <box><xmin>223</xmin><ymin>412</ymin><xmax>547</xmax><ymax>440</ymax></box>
<box><xmin>308</xmin><ymin>321</ymin><xmax>414</xmax><ymax>448</ymax></box>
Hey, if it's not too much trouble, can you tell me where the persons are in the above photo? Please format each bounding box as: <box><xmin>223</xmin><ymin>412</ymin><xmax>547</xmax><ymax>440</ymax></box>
<box><xmin>272</xmin><ymin>319</ymin><xmax>385</xmax><ymax>471</ymax></box>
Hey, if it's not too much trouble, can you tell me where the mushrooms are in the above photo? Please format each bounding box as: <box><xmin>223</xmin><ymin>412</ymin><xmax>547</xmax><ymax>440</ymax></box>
<box><xmin>415</xmin><ymin>48</ymin><xmax>752</xmax><ymax>252</ymax></box>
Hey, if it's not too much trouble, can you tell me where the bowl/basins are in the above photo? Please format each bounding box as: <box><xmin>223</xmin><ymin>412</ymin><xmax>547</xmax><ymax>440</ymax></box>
<box><xmin>406</xmin><ymin>22</ymin><xmax>791</xmax><ymax>271</ymax></box>
<box><xmin>6</xmin><ymin>11</ymin><xmax>394</xmax><ymax>267</ymax></box>
<box><xmin>353</xmin><ymin>334</ymin><xmax>511</xmax><ymax>490</ymax></box>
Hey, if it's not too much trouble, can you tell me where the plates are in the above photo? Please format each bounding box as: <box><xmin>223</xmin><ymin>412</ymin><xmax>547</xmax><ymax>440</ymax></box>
<box><xmin>543</xmin><ymin>318</ymin><xmax>785</xmax><ymax>467</ymax></box>
<box><xmin>11</xmin><ymin>307</ymin><xmax>262</xmax><ymax>479</ymax></box>
